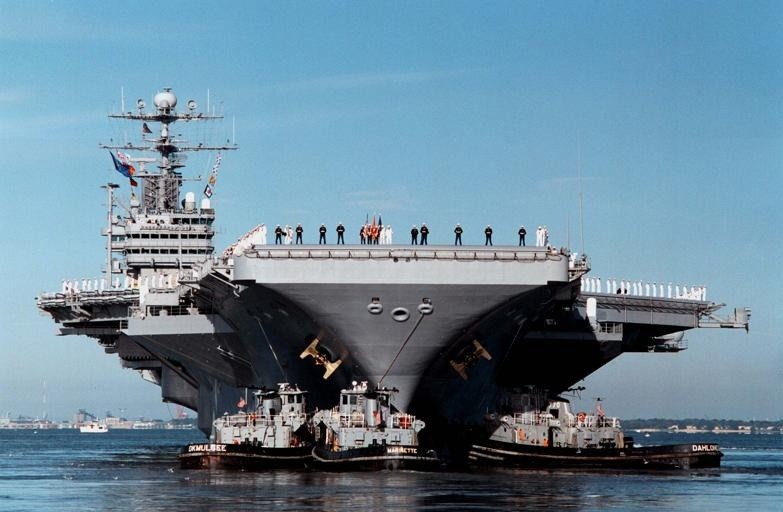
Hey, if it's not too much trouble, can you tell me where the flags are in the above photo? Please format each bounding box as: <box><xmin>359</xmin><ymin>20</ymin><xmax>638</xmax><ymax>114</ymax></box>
<box><xmin>372</xmin><ymin>212</ymin><xmax>376</xmax><ymax>226</ymax></box>
<box><xmin>377</xmin><ymin>214</ymin><xmax>382</xmax><ymax>225</ymax></box>
<box><xmin>364</xmin><ymin>212</ymin><xmax>368</xmax><ymax>225</ymax></box>
<box><xmin>108</xmin><ymin>122</ymin><xmax>223</xmax><ymax>199</ymax></box>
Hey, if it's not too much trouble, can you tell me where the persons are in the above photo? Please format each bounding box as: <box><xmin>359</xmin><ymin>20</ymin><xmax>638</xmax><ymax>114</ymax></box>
<box><xmin>419</xmin><ymin>222</ymin><xmax>429</xmax><ymax>245</ymax></box>
<box><xmin>535</xmin><ymin>224</ymin><xmax>557</xmax><ymax>254</ymax></box>
<box><xmin>318</xmin><ymin>223</ymin><xmax>327</xmax><ymax>244</ymax></box>
<box><xmin>335</xmin><ymin>221</ymin><xmax>345</xmax><ymax>244</ymax></box>
<box><xmin>518</xmin><ymin>224</ymin><xmax>527</xmax><ymax>245</ymax></box>
<box><xmin>453</xmin><ymin>222</ymin><xmax>464</xmax><ymax>246</ymax></box>
<box><xmin>484</xmin><ymin>224</ymin><xmax>493</xmax><ymax>246</ymax></box>
<box><xmin>581</xmin><ymin>277</ymin><xmax>708</xmax><ymax>302</ymax></box>
<box><xmin>359</xmin><ymin>224</ymin><xmax>393</xmax><ymax>245</ymax></box>
<box><xmin>284</xmin><ymin>225</ymin><xmax>293</xmax><ymax>244</ymax></box>
<box><xmin>410</xmin><ymin>224</ymin><xmax>419</xmax><ymax>244</ymax></box>
<box><xmin>60</xmin><ymin>222</ymin><xmax>267</xmax><ymax>296</ymax></box>
<box><xmin>295</xmin><ymin>222</ymin><xmax>303</xmax><ymax>244</ymax></box>
<box><xmin>275</xmin><ymin>223</ymin><xmax>283</xmax><ymax>245</ymax></box>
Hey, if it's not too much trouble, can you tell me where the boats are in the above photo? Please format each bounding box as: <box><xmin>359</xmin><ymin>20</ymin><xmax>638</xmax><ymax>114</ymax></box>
<box><xmin>78</xmin><ymin>417</ymin><xmax>109</xmax><ymax>434</ymax></box>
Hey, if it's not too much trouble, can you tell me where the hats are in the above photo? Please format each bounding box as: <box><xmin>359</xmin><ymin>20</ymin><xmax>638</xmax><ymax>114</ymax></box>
<box><xmin>520</xmin><ymin>225</ymin><xmax>543</xmax><ymax>230</ymax></box>
<box><xmin>320</xmin><ymin>221</ymin><xmax>343</xmax><ymax>227</ymax></box>
<box><xmin>411</xmin><ymin>222</ymin><xmax>427</xmax><ymax>228</ymax></box>
<box><xmin>276</xmin><ymin>221</ymin><xmax>302</xmax><ymax>228</ymax></box>
<box><xmin>457</xmin><ymin>222</ymin><xmax>491</xmax><ymax>228</ymax></box>
<box><xmin>360</xmin><ymin>222</ymin><xmax>393</xmax><ymax>231</ymax></box>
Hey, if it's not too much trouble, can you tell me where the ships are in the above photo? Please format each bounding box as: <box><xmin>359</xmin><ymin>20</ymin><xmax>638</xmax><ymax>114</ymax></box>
<box><xmin>29</xmin><ymin>85</ymin><xmax>756</xmax><ymax>443</ymax></box>
